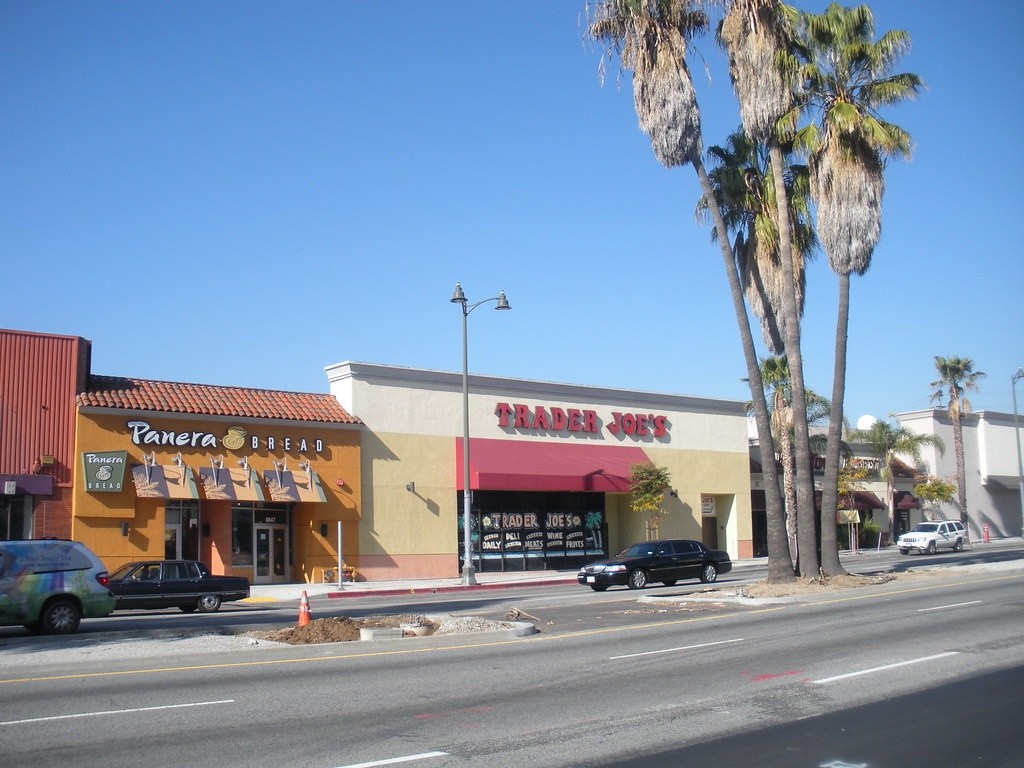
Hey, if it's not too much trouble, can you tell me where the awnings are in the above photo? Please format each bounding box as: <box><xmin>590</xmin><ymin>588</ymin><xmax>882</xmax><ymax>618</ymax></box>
<box><xmin>456</xmin><ymin>437</ymin><xmax>668</xmax><ymax>493</ymax></box>
<box><xmin>130</xmin><ymin>463</ymin><xmax>327</xmax><ymax>503</ymax></box>
<box><xmin>795</xmin><ymin>489</ymin><xmax>922</xmax><ymax>511</ymax></box>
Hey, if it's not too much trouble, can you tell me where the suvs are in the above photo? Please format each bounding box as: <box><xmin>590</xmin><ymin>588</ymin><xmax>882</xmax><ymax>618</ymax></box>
<box><xmin>0</xmin><ymin>536</ymin><xmax>117</xmax><ymax>635</ymax></box>
<box><xmin>897</xmin><ymin>519</ymin><xmax>968</xmax><ymax>556</ymax></box>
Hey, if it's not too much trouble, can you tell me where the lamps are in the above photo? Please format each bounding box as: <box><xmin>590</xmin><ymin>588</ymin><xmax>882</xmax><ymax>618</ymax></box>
<box><xmin>203</xmin><ymin>523</ymin><xmax>211</xmax><ymax>536</ymax></box>
<box><xmin>148</xmin><ymin>451</ymin><xmax>158</xmax><ymax>467</ymax></box>
<box><xmin>277</xmin><ymin>457</ymin><xmax>289</xmax><ymax>471</ymax></box>
<box><xmin>237</xmin><ymin>455</ymin><xmax>248</xmax><ymax>470</ymax></box>
<box><xmin>121</xmin><ymin>522</ymin><xmax>128</xmax><ymax>536</ymax></box>
<box><xmin>321</xmin><ymin>523</ymin><xmax>328</xmax><ymax>537</ymax></box>
<box><xmin>214</xmin><ymin>455</ymin><xmax>225</xmax><ymax>469</ymax></box>
<box><xmin>173</xmin><ymin>453</ymin><xmax>184</xmax><ymax>467</ymax></box>
<box><xmin>300</xmin><ymin>459</ymin><xmax>311</xmax><ymax>473</ymax></box>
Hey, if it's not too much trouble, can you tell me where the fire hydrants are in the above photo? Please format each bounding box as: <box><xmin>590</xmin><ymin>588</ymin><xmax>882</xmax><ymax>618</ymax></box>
<box><xmin>983</xmin><ymin>524</ymin><xmax>989</xmax><ymax>543</ymax></box>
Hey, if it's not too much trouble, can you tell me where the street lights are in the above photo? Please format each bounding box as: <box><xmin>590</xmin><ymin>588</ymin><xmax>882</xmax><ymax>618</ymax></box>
<box><xmin>1010</xmin><ymin>368</ymin><xmax>1024</xmax><ymax>514</ymax></box>
<box><xmin>450</xmin><ymin>278</ymin><xmax>511</xmax><ymax>582</ymax></box>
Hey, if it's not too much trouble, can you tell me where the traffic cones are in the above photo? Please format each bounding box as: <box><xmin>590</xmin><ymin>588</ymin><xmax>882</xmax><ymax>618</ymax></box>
<box><xmin>297</xmin><ymin>590</ymin><xmax>314</xmax><ymax>630</ymax></box>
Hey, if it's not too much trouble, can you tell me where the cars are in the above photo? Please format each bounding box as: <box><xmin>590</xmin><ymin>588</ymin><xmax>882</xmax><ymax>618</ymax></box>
<box><xmin>577</xmin><ymin>539</ymin><xmax>733</xmax><ymax>591</ymax></box>
<box><xmin>98</xmin><ymin>559</ymin><xmax>251</xmax><ymax>614</ymax></box>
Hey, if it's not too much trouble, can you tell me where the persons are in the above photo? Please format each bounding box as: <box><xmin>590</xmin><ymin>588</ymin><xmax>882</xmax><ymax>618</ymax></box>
<box><xmin>139</xmin><ymin>568</ymin><xmax>159</xmax><ymax>580</ymax></box>
